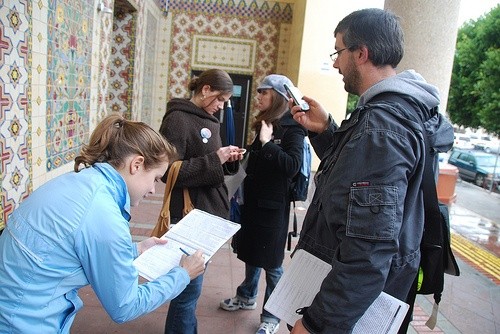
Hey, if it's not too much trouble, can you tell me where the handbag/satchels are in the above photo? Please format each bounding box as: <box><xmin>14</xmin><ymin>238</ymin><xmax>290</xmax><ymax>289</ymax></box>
<box><xmin>417</xmin><ymin>202</ymin><xmax>460</xmax><ymax>295</ymax></box>
<box><xmin>150</xmin><ymin>161</ymin><xmax>195</xmax><ymax>238</ymax></box>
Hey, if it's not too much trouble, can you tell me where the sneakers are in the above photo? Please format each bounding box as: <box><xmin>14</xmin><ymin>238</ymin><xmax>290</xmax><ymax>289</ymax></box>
<box><xmin>220</xmin><ymin>296</ymin><xmax>257</xmax><ymax>311</ymax></box>
<box><xmin>255</xmin><ymin>322</ymin><xmax>279</xmax><ymax>334</ymax></box>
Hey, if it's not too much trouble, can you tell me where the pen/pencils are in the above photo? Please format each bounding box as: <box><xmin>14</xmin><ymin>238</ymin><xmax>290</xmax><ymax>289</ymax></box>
<box><xmin>179</xmin><ymin>247</ymin><xmax>190</xmax><ymax>256</ymax></box>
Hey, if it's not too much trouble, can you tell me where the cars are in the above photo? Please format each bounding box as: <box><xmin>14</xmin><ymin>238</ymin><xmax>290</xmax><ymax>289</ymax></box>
<box><xmin>437</xmin><ymin>124</ymin><xmax>500</xmax><ymax>165</ymax></box>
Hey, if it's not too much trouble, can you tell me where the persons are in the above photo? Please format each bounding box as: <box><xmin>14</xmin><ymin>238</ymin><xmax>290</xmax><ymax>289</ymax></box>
<box><xmin>391</xmin><ymin>69</ymin><xmax>458</xmax><ymax>334</ymax></box>
<box><xmin>288</xmin><ymin>7</ymin><xmax>441</xmax><ymax>334</ymax></box>
<box><xmin>219</xmin><ymin>74</ymin><xmax>310</xmax><ymax>334</ymax></box>
<box><xmin>0</xmin><ymin>115</ymin><xmax>210</xmax><ymax>334</ymax></box>
<box><xmin>149</xmin><ymin>68</ymin><xmax>244</xmax><ymax>334</ymax></box>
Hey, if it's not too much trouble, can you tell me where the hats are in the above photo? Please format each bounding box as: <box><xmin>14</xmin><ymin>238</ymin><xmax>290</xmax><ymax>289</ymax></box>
<box><xmin>257</xmin><ymin>74</ymin><xmax>293</xmax><ymax>102</ymax></box>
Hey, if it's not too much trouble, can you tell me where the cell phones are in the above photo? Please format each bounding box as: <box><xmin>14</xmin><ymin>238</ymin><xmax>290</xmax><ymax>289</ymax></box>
<box><xmin>283</xmin><ymin>84</ymin><xmax>310</xmax><ymax>111</ymax></box>
<box><xmin>230</xmin><ymin>149</ymin><xmax>247</xmax><ymax>155</ymax></box>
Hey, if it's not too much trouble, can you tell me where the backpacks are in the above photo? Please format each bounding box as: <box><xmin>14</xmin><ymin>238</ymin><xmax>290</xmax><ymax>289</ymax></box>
<box><xmin>280</xmin><ymin>125</ymin><xmax>311</xmax><ymax>201</ymax></box>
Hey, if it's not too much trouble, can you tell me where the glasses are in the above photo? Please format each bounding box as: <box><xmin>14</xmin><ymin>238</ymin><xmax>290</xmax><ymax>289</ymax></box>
<box><xmin>330</xmin><ymin>47</ymin><xmax>349</xmax><ymax>62</ymax></box>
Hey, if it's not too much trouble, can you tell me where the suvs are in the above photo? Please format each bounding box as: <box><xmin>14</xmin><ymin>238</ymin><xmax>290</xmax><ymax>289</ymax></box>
<box><xmin>448</xmin><ymin>147</ymin><xmax>500</xmax><ymax>189</ymax></box>
